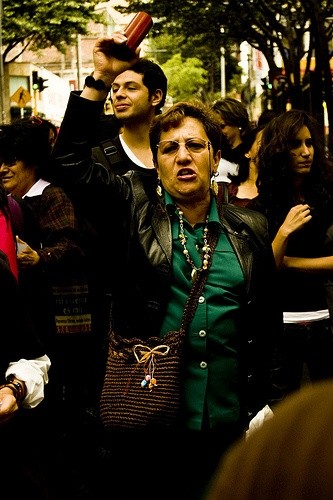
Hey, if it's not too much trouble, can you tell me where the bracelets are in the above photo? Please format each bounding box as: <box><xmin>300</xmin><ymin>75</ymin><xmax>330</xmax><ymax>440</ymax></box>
<box><xmin>85</xmin><ymin>76</ymin><xmax>112</xmax><ymax>92</ymax></box>
<box><xmin>0</xmin><ymin>378</ymin><xmax>23</xmax><ymax>409</ymax></box>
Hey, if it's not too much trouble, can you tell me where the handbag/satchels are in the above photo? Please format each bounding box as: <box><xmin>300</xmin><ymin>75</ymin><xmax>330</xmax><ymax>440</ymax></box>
<box><xmin>100</xmin><ymin>329</ymin><xmax>183</xmax><ymax>429</ymax></box>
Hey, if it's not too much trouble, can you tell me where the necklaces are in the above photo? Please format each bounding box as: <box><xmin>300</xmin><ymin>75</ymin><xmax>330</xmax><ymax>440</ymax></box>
<box><xmin>174</xmin><ymin>203</ymin><xmax>212</xmax><ymax>281</ymax></box>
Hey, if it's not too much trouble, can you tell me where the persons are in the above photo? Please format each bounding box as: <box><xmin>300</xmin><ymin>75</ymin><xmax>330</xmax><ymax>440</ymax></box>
<box><xmin>48</xmin><ymin>11</ymin><xmax>291</xmax><ymax>500</ymax></box>
<box><xmin>0</xmin><ymin>98</ymin><xmax>333</xmax><ymax>500</ymax></box>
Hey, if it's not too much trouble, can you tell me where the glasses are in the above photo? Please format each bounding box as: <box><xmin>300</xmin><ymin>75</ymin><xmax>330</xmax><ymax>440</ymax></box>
<box><xmin>0</xmin><ymin>155</ymin><xmax>21</xmax><ymax>165</ymax></box>
<box><xmin>155</xmin><ymin>138</ymin><xmax>209</xmax><ymax>156</ymax></box>
